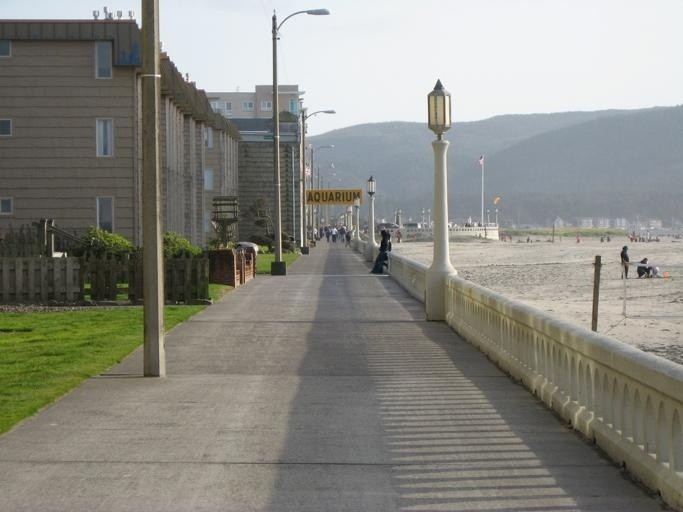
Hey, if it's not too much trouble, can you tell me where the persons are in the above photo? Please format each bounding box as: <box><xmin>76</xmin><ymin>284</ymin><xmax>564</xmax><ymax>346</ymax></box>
<box><xmin>628</xmin><ymin>230</ymin><xmax>660</xmax><ymax>243</ymax></box>
<box><xmin>312</xmin><ymin>221</ymin><xmax>355</xmax><ymax>249</ymax></box>
<box><xmin>370</xmin><ymin>229</ymin><xmax>388</xmax><ymax>275</ymax></box>
<box><xmin>383</xmin><ymin>232</ymin><xmax>392</xmax><ymax>250</ymax></box>
<box><xmin>619</xmin><ymin>245</ymin><xmax>632</xmax><ymax>280</ymax></box>
<box><xmin>637</xmin><ymin>257</ymin><xmax>649</xmax><ymax>280</ymax></box>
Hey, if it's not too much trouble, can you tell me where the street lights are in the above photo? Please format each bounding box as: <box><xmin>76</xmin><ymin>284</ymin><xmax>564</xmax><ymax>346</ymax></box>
<box><xmin>271</xmin><ymin>8</ymin><xmax>342</xmax><ymax>261</ymax></box>
<box><xmin>346</xmin><ymin>176</ymin><xmax>375</xmax><ymax>242</ymax></box>
<box><xmin>426</xmin><ymin>80</ymin><xmax>453</xmax><ymax>267</ymax></box>
